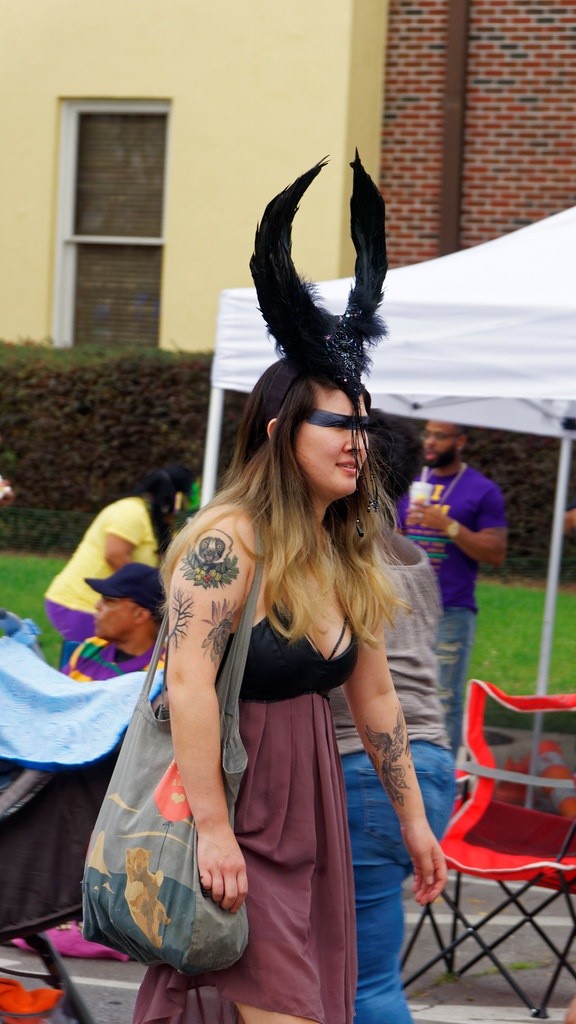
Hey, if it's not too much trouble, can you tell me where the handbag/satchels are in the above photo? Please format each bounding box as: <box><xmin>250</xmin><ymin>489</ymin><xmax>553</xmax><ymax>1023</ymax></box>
<box><xmin>83</xmin><ymin>699</ymin><xmax>248</xmax><ymax>976</ymax></box>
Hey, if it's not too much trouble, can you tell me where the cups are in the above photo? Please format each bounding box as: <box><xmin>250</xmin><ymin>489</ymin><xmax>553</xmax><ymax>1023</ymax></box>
<box><xmin>408</xmin><ymin>480</ymin><xmax>434</xmax><ymax>510</ymax></box>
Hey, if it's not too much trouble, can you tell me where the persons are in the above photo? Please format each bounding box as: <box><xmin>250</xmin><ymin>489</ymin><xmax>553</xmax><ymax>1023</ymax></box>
<box><xmin>44</xmin><ymin>466</ymin><xmax>192</xmax><ymax>684</ymax></box>
<box><xmin>131</xmin><ymin>359</ymin><xmax>457</xmax><ymax>1024</ymax></box>
<box><xmin>395</xmin><ymin>420</ymin><xmax>508</xmax><ymax>760</ymax></box>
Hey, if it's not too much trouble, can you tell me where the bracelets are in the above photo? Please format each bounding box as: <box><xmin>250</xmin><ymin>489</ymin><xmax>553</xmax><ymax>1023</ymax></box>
<box><xmin>447</xmin><ymin>518</ymin><xmax>460</xmax><ymax>537</ymax></box>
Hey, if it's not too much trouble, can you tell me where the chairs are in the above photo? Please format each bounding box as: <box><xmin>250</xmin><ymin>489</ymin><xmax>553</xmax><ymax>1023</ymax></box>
<box><xmin>1</xmin><ymin>636</ymin><xmax>169</xmax><ymax>1024</ymax></box>
<box><xmin>400</xmin><ymin>680</ymin><xmax>576</xmax><ymax>1018</ymax></box>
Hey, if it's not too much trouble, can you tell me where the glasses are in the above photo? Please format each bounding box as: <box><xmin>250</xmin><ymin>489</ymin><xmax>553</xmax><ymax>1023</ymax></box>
<box><xmin>420</xmin><ymin>429</ymin><xmax>463</xmax><ymax>444</ymax></box>
<box><xmin>101</xmin><ymin>595</ymin><xmax>135</xmax><ymax>608</ymax></box>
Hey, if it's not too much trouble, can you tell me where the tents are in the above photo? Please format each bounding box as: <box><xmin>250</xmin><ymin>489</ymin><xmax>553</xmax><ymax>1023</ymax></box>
<box><xmin>199</xmin><ymin>203</ymin><xmax>576</xmax><ymax>811</ymax></box>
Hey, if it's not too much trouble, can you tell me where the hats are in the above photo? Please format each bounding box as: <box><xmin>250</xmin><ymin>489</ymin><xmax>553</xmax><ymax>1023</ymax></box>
<box><xmin>84</xmin><ymin>562</ymin><xmax>165</xmax><ymax>612</ymax></box>
